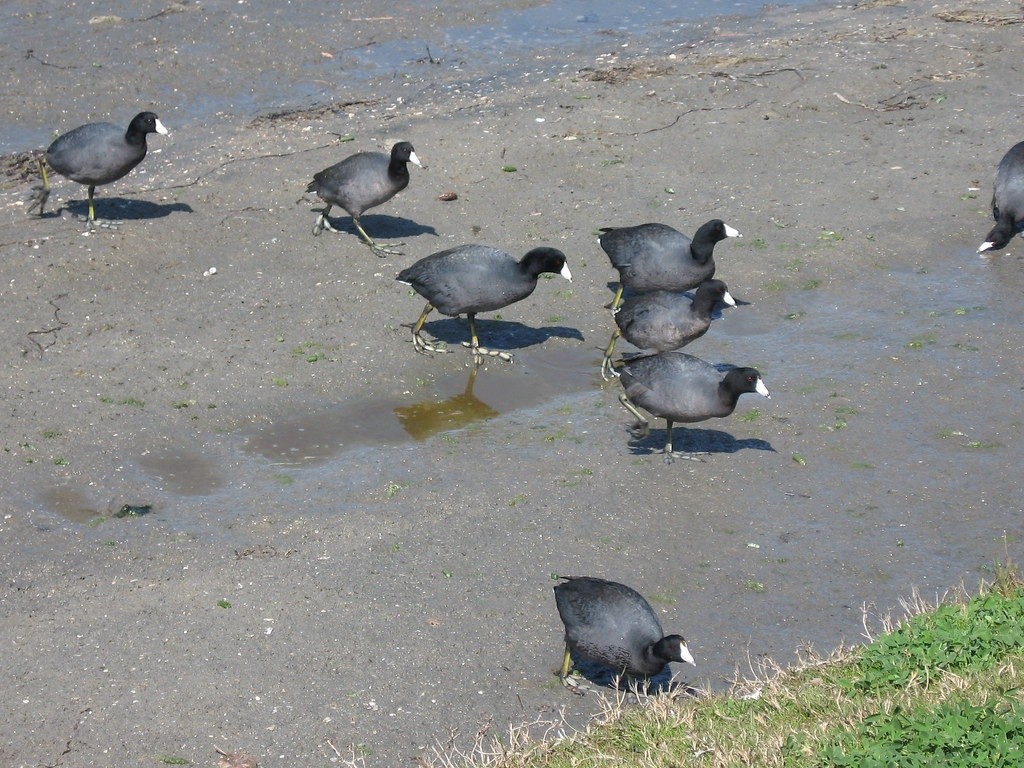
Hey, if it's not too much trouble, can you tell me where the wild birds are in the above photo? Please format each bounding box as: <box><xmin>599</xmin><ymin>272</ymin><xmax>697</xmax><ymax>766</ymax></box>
<box><xmin>595</xmin><ymin>218</ymin><xmax>773</xmax><ymax>464</ymax></box>
<box><xmin>20</xmin><ymin>112</ymin><xmax>171</xmax><ymax>234</ymax></box>
<box><xmin>305</xmin><ymin>141</ymin><xmax>424</xmax><ymax>258</ymax></box>
<box><xmin>394</xmin><ymin>243</ymin><xmax>574</xmax><ymax>366</ymax></box>
<box><xmin>549</xmin><ymin>574</ymin><xmax>696</xmax><ymax>695</ymax></box>
<box><xmin>976</xmin><ymin>139</ymin><xmax>1024</xmax><ymax>255</ymax></box>
